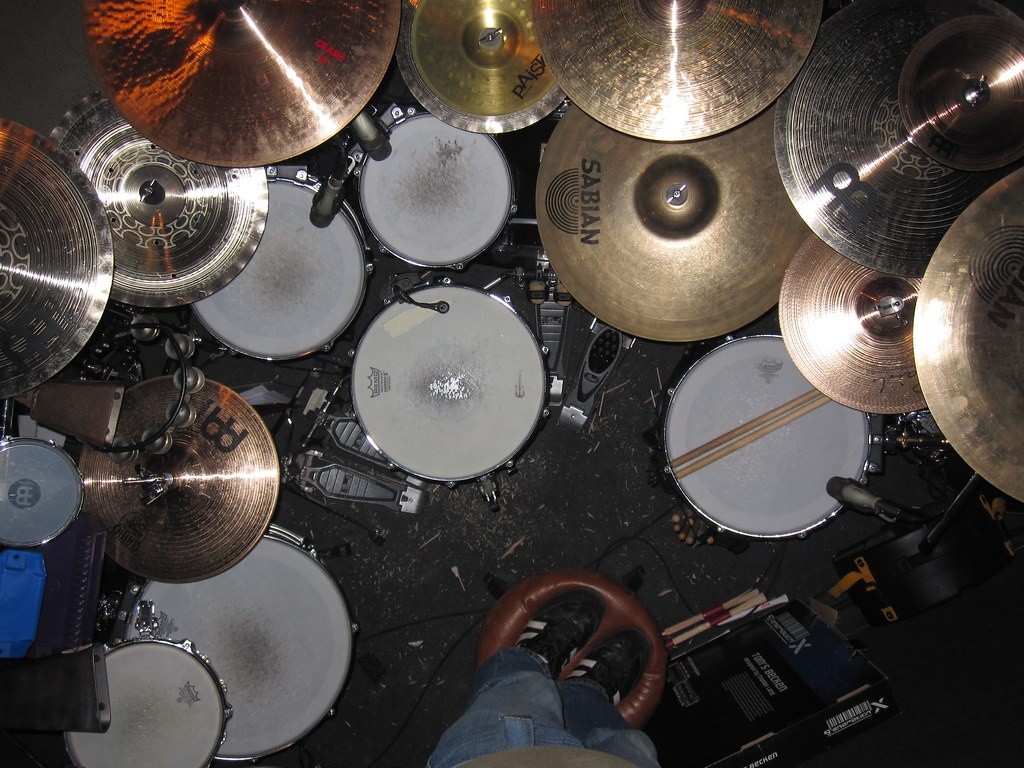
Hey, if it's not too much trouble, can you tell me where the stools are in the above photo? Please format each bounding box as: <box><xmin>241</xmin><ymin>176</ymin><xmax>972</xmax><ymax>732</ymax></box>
<box><xmin>485</xmin><ymin>565</ymin><xmax>667</xmax><ymax>728</ymax></box>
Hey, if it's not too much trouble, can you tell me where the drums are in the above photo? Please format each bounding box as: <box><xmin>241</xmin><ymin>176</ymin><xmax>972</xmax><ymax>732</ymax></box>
<box><xmin>355</xmin><ymin>284</ymin><xmax>545</xmax><ymax>481</ymax></box>
<box><xmin>359</xmin><ymin>114</ymin><xmax>511</xmax><ymax>270</ymax></box>
<box><xmin>103</xmin><ymin>524</ymin><xmax>352</xmax><ymax>761</ymax></box>
<box><xmin>63</xmin><ymin>640</ymin><xmax>234</xmax><ymax>767</ymax></box>
<box><xmin>194</xmin><ymin>169</ymin><xmax>369</xmax><ymax>355</ymax></box>
<box><xmin>663</xmin><ymin>335</ymin><xmax>883</xmax><ymax>538</ymax></box>
<box><xmin>0</xmin><ymin>435</ymin><xmax>83</xmax><ymax>549</ymax></box>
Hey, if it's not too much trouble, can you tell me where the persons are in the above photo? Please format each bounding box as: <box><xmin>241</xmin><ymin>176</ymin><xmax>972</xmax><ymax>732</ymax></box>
<box><xmin>427</xmin><ymin>599</ymin><xmax>660</xmax><ymax>768</ymax></box>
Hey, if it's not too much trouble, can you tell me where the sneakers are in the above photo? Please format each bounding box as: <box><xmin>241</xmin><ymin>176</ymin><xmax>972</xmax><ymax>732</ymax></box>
<box><xmin>515</xmin><ymin>587</ymin><xmax>605</xmax><ymax>677</ymax></box>
<box><xmin>566</xmin><ymin>625</ymin><xmax>653</xmax><ymax>711</ymax></box>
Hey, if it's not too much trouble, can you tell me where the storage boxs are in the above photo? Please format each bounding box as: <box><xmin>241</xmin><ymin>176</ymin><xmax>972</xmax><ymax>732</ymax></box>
<box><xmin>646</xmin><ymin>595</ymin><xmax>904</xmax><ymax>768</ymax></box>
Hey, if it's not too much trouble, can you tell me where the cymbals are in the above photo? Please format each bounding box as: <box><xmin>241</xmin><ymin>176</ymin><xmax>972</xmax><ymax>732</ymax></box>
<box><xmin>46</xmin><ymin>93</ymin><xmax>268</xmax><ymax>310</ymax></box>
<box><xmin>911</xmin><ymin>173</ymin><xmax>1024</xmax><ymax>500</ymax></box>
<box><xmin>78</xmin><ymin>375</ymin><xmax>280</xmax><ymax>582</ymax></box>
<box><xmin>1</xmin><ymin>124</ymin><xmax>114</xmax><ymax>394</ymax></box>
<box><xmin>397</xmin><ymin>0</ymin><xmax>567</xmax><ymax>134</ymax></box>
<box><xmin>87</xmin><ymin>1</ymin><xmax>400</xmax><ymax>167</ymax></box>
<box><xmin>778</xmin><ymin>235</ymin><xmax>930</xmax><ymax>414</ymax></box>
<box><xmin>532</xmin><ymin>0</ymin><xmax>825</xmax><ymax>142</ymax></box>
<box><xmin>899</xmin><ymin>16</ymin><xmax>1024</xmax><ymax>168</ymax></box>
<box><xmin>535</xmin><ymin>103</ymin><xmax>812</xmax><ymax>343</ymax></box>
<box><xmin>774</xmin><ymin>0</ymin><xmax>1013</xmax><ymax>273</ymax></box>
